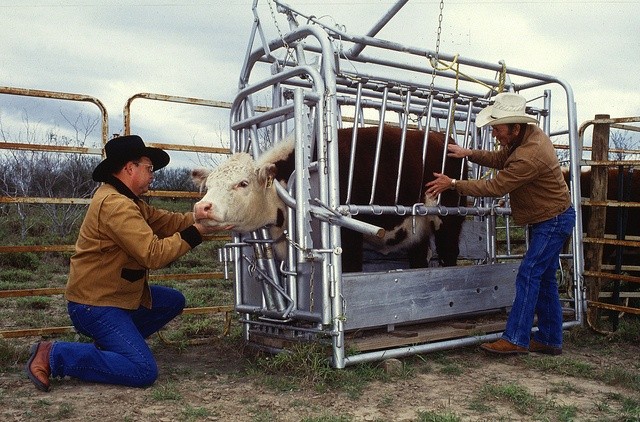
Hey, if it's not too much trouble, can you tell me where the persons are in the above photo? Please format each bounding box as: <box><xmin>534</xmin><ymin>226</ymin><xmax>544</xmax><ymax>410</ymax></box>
<box><xmin>424</xmin><ymin>92</ymin><xmax>576</xmax><ymax>356</ymax></box>
<box><xmin>25</xmin><ymin>135</ymin><xmax>239</xmax><ymax>393</ymax></box>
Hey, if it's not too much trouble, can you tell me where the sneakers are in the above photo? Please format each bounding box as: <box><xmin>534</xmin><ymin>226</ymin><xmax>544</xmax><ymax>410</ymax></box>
<box><xmin>480</xmin><ymin>339</ymin><xmax>529</xmax><ymax>356</ymax></box>
<box><xmin>26</xmin><ymin>341</ymin><xmax>57</xmax><ymax>391</ymax></box>
<box><xmin>529</xmin><ymin>338</ymin><xmax>563</xmax><ymax>354</ymax></box>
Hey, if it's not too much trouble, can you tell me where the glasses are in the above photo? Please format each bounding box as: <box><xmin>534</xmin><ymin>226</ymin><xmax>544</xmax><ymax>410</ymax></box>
<box><xmin>129</xmin><ymin>160</ymin><xmax>154</xmax><ymax>173</ymax></box>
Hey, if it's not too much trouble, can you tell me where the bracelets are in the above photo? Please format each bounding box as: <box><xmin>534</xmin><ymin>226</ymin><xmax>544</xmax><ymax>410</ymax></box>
<box><xmin>450</xmin><ymin>178</ymin><xmax>456</xmax><ymax>191</ymax></box>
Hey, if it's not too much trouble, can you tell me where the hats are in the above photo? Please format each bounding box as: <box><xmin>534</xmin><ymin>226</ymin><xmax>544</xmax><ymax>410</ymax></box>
<box><xmin>475</xmin><ymin>93</ymin><xmax>541</xmax><ymax>128</ymax></box>
<box><xmin>92</xmin><ymin>135</ymin><xmax>170</xmax><ymax>182</ymax></box>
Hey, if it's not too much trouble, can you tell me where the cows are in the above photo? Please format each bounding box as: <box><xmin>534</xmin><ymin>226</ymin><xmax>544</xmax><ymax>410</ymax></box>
<box><xmin>192</xmin><ymin>126</ymin><xmax>469</xmax><ymax>272</ymax></box>
<box><xmin>558</xmin><ymin>166</ymin><xmax>639</xmax><ymax>292</ymax></box>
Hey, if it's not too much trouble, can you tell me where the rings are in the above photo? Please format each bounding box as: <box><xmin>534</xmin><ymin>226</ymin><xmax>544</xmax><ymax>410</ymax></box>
<box><xmin>433</xmin><ymin>190</ymin><xmax>437</xmax><ymax>193</ymax></box>
